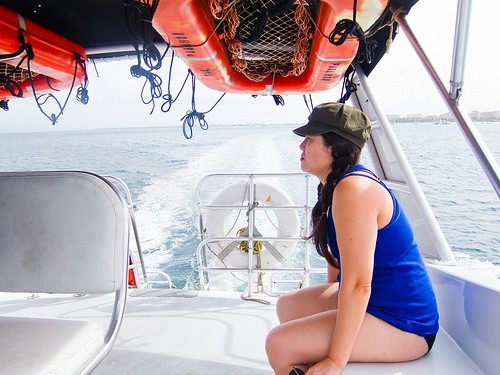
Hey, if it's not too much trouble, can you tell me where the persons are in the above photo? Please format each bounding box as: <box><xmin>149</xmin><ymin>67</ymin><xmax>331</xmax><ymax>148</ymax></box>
<box><xmin>265</xmin><ymin>102</ymin><xmax>439</xmax><ymax>375</ymax></box>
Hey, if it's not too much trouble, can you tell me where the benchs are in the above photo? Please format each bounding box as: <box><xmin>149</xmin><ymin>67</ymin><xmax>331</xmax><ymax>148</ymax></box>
<box><xmin>1</xmin><ymin>170</ymin><xmax>132</xmax><ymax>375</ymax></box>
<box><xmin>316</xmin><ymin>262</ymin><xmax>499</xmax><ymax>373</ymax></box>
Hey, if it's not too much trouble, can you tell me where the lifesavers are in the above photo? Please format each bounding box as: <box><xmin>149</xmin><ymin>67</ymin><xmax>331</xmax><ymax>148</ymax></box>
<box><xmin>205</xmin><ymin>180</ymin><xmax>300</xmax><ymax>269</ymax></box>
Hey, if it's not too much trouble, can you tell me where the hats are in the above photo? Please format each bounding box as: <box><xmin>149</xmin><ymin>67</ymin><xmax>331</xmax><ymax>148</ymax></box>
<box><xmin>293</xmin><ymin>102</ymin><xmax>372</xmax><ymax>149</ymax></box>
